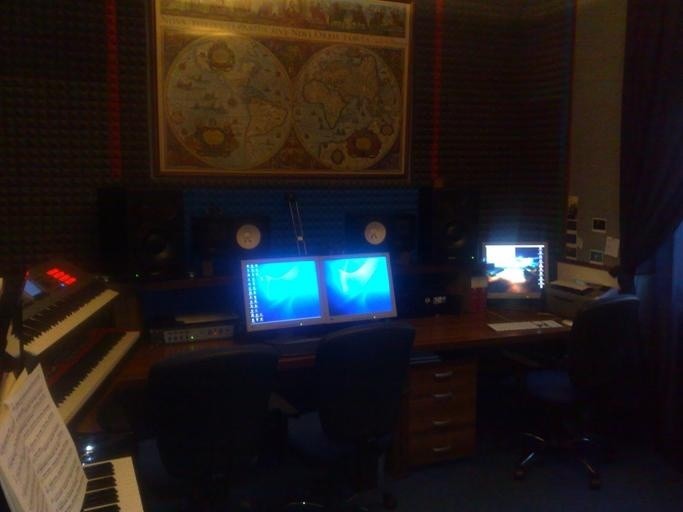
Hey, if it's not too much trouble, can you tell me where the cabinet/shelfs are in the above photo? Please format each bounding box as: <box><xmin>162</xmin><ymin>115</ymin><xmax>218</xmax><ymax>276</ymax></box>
<box><xmin>390</xmin><ymin>356</ymin><xmax>478</xmax><ymax>482</ymax></box>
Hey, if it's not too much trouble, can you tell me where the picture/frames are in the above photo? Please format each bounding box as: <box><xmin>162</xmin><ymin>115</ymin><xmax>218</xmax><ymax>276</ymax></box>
<box><xmin>146</xmin><ymin>0</ymin><xmax>414</xmax><ymax>181</ymax></box>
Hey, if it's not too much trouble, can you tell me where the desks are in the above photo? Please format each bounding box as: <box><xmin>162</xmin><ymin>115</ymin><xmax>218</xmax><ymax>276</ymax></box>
<box><xmin>75</xmin><ymin>305</ymin><xmax>571</xmax><ymax>436</ymax></box>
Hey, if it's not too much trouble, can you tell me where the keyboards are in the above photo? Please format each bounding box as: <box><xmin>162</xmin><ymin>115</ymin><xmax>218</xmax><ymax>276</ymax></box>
<box><xmin>487</xmin><ymin>320</ymin><xmax>563</xmax><ymax>332</ymax></box>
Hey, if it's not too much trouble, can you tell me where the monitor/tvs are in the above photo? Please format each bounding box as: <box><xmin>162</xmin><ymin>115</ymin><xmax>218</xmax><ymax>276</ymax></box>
<box><xmin>318</xmin><ymin>251</ymin><xmax>399</xmax><ymax>339</ymax></box>
<box><xmin>481</xmin><ymin>241</ymin><xmax>549</xmax><ymax>300</ymax></box>
<box><xmin>241</xmin><ymin>256</ymin><xmax>327</xmax><ymax>345</ymax></box>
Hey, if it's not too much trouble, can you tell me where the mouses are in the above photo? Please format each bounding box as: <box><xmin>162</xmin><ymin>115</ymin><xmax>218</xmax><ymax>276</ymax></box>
<box><xmin>562</xmin><ymin>320</ymin><xmax>574</xmax><ymax>327</ymax></box>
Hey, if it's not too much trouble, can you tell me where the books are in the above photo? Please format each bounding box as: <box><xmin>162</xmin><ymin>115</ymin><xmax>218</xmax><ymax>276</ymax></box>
<box><xmin>0</xmin><ymin>362</ymin><xmax>88</xmax><ymax>511</ymax></box>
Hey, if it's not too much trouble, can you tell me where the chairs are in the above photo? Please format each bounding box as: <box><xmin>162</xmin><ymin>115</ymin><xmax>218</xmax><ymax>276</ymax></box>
<box><xmin>492</xmin><ymin>295</ymin><xmax>642</xmax><ymax>491</ymax></box>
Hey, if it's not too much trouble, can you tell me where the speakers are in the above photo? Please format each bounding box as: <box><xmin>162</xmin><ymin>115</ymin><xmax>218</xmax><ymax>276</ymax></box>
<box><xmin>418</xmin><ymin>187</ymin><xmax>480</xmax><ymax>265</ymax></box>
<box><xmin>214</xmin><ymin>213</ymin><xmax>270</xmax><ymax>277</ymax></box>
<box><xmin>346</xmin><ymin>210</ymin><xmax>397</xmax><ymax>271</ymax></box>
<box><xmin>97</xmin><ymin>185</ymin><xmax>184</xmax><ymax>287</ymax></box>
